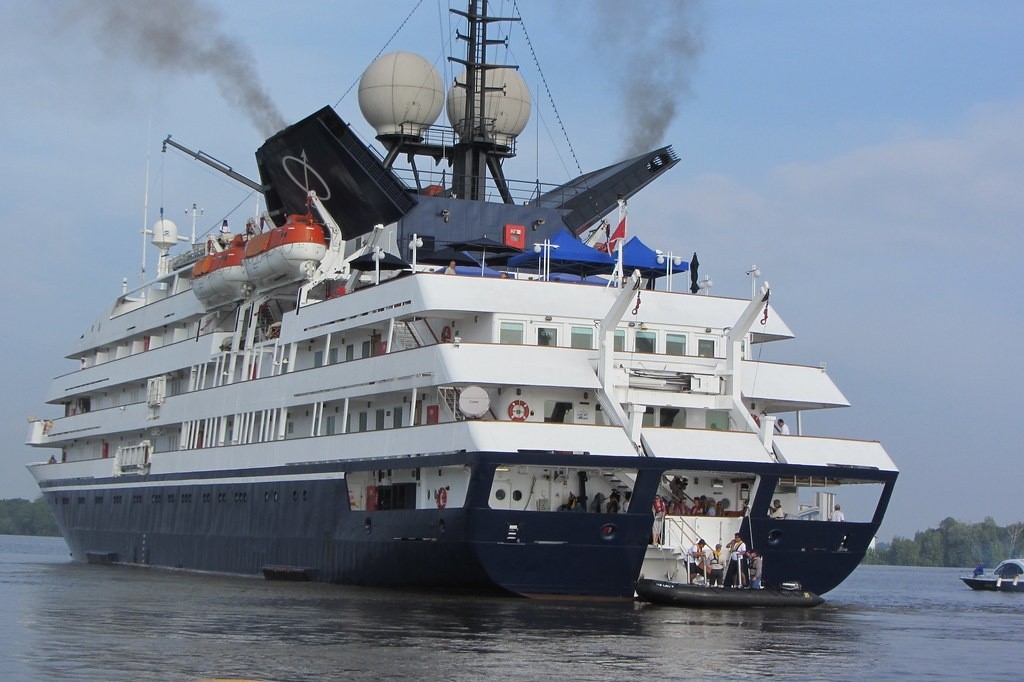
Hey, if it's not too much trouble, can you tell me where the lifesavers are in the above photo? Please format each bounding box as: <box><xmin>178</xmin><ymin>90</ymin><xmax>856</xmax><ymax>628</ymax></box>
<box><xmin>750</xmin><ymin>413</ymin><xmax>760</xmax><ymax>427</ymax></box>
<box><xmin>441</xmin><ymin>326</ymin><xmax>451</xmax><ymax>343</ymax></box>
<box><xmin>437</xmin><ymin>488</ymin><xmax>446</xmax><ymax>508</ymax></box>
<box><xmin>509</xmin><ymin>400</ymin><xmax>530</xmax><ymax>422</ymax></box>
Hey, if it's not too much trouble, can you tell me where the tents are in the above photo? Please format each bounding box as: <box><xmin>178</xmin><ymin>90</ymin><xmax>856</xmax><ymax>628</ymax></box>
<box><xmin>506</xmin><ymin>228</ymin><xmax>617</xmax><ymax>286</ymax></box>
<box><xmin>534</xmin><ymin>273</ymin><xmax>618</xmax><ymax>287</ymax></box>
<box><xmin>612</xmin><ymin>235</ymin><xmax>689</xmax><ymax>294</ymax></box>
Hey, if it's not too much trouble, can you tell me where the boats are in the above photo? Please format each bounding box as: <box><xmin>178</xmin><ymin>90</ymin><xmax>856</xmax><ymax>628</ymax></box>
<box><xmin>957</xmin><ymin>559</ymin><xmax>1024</xmax><ymax>592</ymax></box>
<box><xmin>189</xmin><ymin>214</ymin><xmax>327</xmax><ymax>309</ymax></box>
<box><xmin>631</xmin><ymin>577</ymin><xmax>826</xmax><ymax>609</ymax></box>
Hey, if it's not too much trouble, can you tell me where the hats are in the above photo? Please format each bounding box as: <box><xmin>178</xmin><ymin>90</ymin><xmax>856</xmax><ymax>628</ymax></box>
<box><xmin>698</xmin><ymin>539</ymin><xmax>706</xmax><ymax>544</ymax></box>
<box><xmin>700</xmin><ymin>496</ymin><xmax>708</xmax><ymax>500</ymax></box>
<box><xmin>835</xmin><ymin>504</ymin><xmax>840</xmax><ymax>509</ymax></box>
<box><xmin>716</xmin><ymin>544</ymin><xmax>722</xmax><ymax>549</ymax></box>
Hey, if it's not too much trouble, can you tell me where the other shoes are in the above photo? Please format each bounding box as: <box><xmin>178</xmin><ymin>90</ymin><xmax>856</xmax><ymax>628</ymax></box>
<box><xmin>653</xmin><ymin>542</ymin><xmax>658</xmax><ymax>546</ymax></box>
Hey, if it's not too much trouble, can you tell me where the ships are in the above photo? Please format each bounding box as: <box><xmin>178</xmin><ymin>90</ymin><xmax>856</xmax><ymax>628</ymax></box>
<box><xmin>22</xmin><ymin>0</ymin><xmax>900</xmax><ymax>597</ymax></box>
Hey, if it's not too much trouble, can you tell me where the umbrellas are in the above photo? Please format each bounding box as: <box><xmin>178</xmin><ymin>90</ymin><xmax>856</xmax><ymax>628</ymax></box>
<box><xmin>690</xmin><ymin>252</ymin><xmax>700</xmax><ymax>294</ymax></box>
<box><xmin>349</xmin><ymin>249</ymin><xmax>410</xmax><ymax>284</ymax></box>
<box><xmin>417</xmin><ymin>235</ymin><xmax>523</xmax><ymax>277</ymax></box>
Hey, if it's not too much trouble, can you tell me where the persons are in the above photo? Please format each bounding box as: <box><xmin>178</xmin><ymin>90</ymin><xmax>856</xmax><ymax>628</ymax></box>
<box><xmin>707</xmin><ymin>544</ymin><xmax>726</xmax><ymax>589</ymax></box>
<box><xmin>445</xmin><ymin>260</ymin><xmax>456</xmax><ymax>274</ymax></box>
<box><xmin>768</xmin><ymin>500</ymin><xmax>784</xmax><ymax>520</ymax></box>
<box><xmin>652</xmin><ymin>495</ymin><xmax>665</xmax><ymax>545</ymax></box>
<box><xmin>684</xmin><ymin>539</ymin><xmax>710</xmax><ymax>584</ymax></box>
<box><xmin>48</xmin><ymin>455</ymin><xmax>57</xmax><ymax>464</ymax></box>
<box><xmin>667</xmin><ymin>495</ymin><xmax>730</xmax><ymax>517</ymax></box>
<box><xmin>831</xmin><ymin>505</ymin><xmax>844</xmax><ymax>522</ymax></box>
<box><xmin>721</xmin><ymin>533</ymin><xmax>761</xmax><ymax>589</ymax></box>
<box><xmin>778</xmin><ymin>419</ymin><xmax>789</xmax><ymax>435</ymax></box>
<box><xmin>220</xmin><ymin>219</ymin><xmax>230</xmax><ymax>233</ymax></box>
